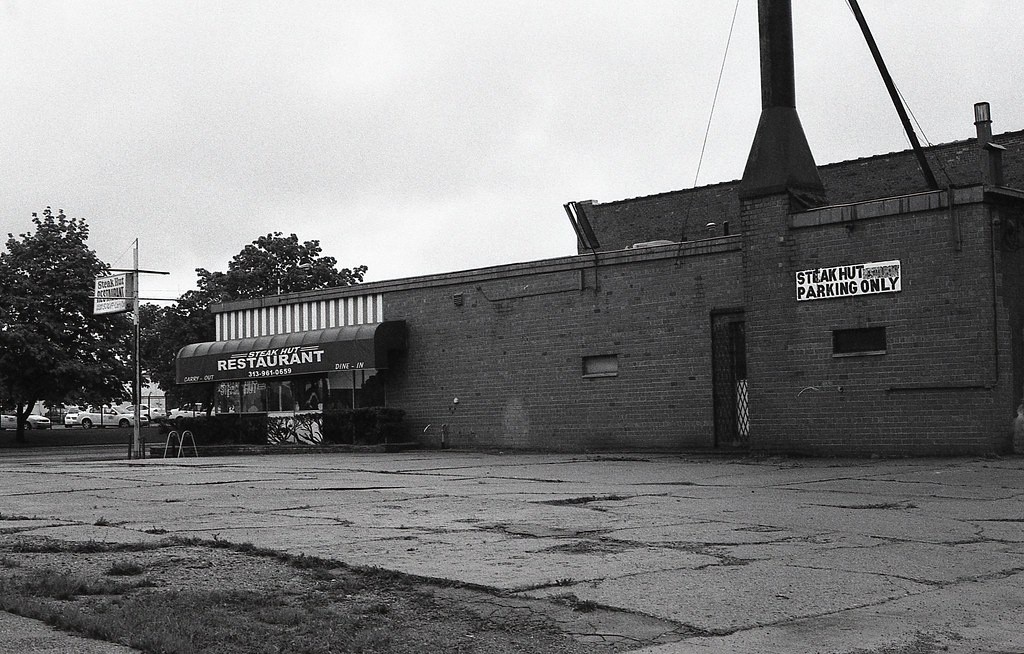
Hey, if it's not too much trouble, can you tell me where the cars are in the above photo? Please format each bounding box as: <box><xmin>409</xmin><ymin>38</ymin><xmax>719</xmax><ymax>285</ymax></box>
<box><xmin>168</xmin><ymin>402</ymin><xmax>236</xmax><ymax>422</ymax></box>
<box><xmin>63</xmin><ymin>406</ymin><xmax>80</xmax><ymax>429</ymax></box>
<box><xmin>125</xmin><ymin>404</ymin><xmax>167</xmax><ymax>423</ymax></box>
<box><xmin>77</xmin><ymin>405</ymin><xmax>149</xmax><ymax>430</ymax></box>
<box><xmin>10</xmin><ymin>410</ymin><xmax>50</xmax><ymax>431</ymax></box>
<box><xmin>0</xmin><ymin>410</ymin><xmax>17</xmax><ymax>430</ymax></box>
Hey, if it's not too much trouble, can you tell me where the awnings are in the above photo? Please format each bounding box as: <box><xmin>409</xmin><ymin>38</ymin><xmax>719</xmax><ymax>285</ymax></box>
<box><xmin>175</xmin><ymin>319</ymin><xmax>406</xmax><ymax>385</ymax></box>
